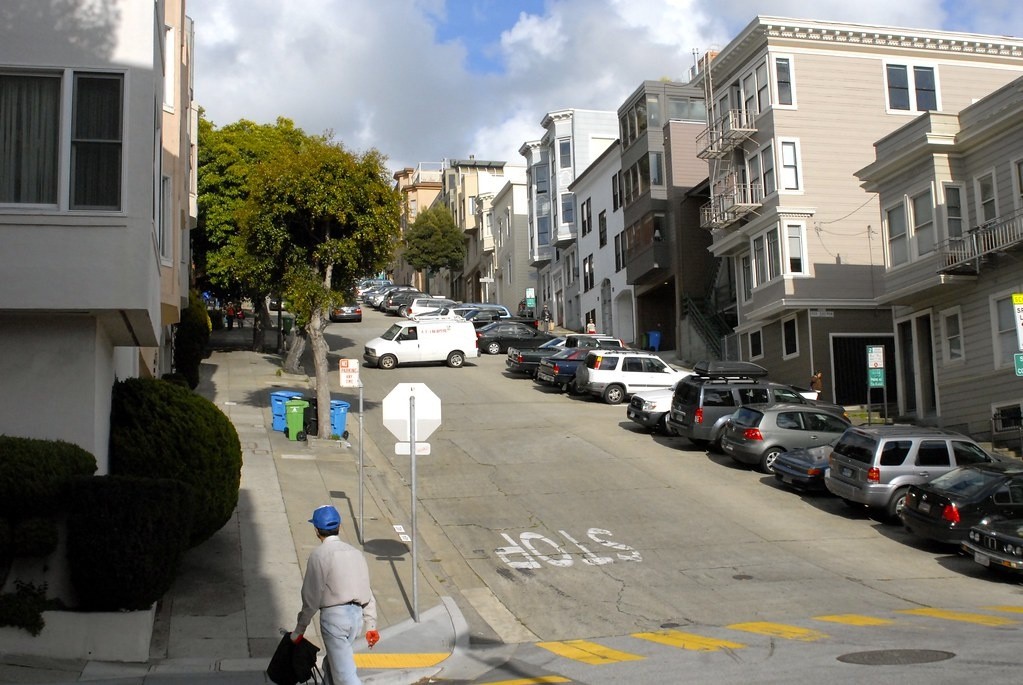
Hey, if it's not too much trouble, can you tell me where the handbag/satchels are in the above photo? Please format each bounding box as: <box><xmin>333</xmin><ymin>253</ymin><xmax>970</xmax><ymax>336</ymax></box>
<box><xmin>266</xmin><ymin>631</ymin><xmax>320</xmax><ymax>685</ymax></box>
<box><xmin>549</xmin><ymin>320</ymin><xmax>555</xmax><ymax>330</ymax></box>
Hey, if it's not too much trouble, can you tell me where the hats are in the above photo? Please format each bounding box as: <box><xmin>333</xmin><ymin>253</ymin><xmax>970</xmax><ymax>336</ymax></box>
<box><xmin>228</xmin><ymin>302</ymin><xmax>232</xmax><ymax>304</ymax></box>
<box><xmin>308</xmin><ymin>504</ymin><xmax>341</xmax><ymax>529</ymax></box>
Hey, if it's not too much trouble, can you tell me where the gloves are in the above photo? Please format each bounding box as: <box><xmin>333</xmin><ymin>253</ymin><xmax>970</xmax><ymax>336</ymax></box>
<box><xmin>290</xmin><ymin>631</ymin><xmax>303</xmax><ymax>644</ymax></box>
<box><xmin>366</xmin><ymin>630</ymin><xmax>379</xmax><ymax>649</ymax></box>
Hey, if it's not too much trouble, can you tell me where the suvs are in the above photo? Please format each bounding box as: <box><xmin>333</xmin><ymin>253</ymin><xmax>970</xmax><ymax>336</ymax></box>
<box><xmin>720</xmin><ymin>401</ymin><xmax>863</xmax><ymax>476</ymax></box>
<box><xmin>823</xmin><ymin>421</ymin><xmax>1018</xmax><ymax>526</ymax></box>
<box><xmin>665</xmin><ymin>361</ymin><xmax>850</xmax><ymax>455</ymax></box>
<box><xmin>575</xmin><ymin>350</ymin><xmax>700</xmax><ymax>406</ymax></box>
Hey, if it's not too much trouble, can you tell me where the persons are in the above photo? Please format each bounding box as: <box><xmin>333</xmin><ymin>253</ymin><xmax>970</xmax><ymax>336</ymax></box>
<box><xmin>809</xmin><ymin>369</ymin><xmax>825</xmax><ymax>400</ymax></box>
<box><xmin>290</xmin><ymin>504</ymin><xmax>380</xmax><ymax>685</ymax></box>
<box><xmin>221</xmin><ymin>299</ymin><xmax>245</xmax><ymax>331</ymax></box>
<box><xmin>587</xmin><ymin>318</ymin><xmax>597</xmax><ymax>334</ymax></box>
<box><xmin>541</xmin><ymin>304</ymin><xmax>554</xmax><ymax>335</ymax></box>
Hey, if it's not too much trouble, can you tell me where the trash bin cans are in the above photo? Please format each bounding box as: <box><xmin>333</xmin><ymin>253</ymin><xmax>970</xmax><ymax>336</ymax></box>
<box><xmin>270</xmin><ymin>391</ymin><xmax>305</xmax><ymax>432</ymax></box>
<box><xmin>331</xmin><ymin>400</ymin><xmax>349</xmax><ymax>440</ymax></box>
<box><xmin>647</xmin><ymin>331</ymin><xmax>661</xmax><ymax>352</ymax></box>
<box><xmin>641</xmin><ymin>333</ymin><xmax>648</xmax><ymax>350</ymax></box>
<box><xmin>282</xmin><ymin>317</ymin><xmax>292</xmax><ymax>335</ymax></box>
<box><xmin>303</xmin><ymin>397</ymin><xmax>318</xmax><ymax>436</ymax></box>
<box><xmin>285</xmin><ymin>400</ymin><xmax>310</xmax><ymax>442</ymax></box>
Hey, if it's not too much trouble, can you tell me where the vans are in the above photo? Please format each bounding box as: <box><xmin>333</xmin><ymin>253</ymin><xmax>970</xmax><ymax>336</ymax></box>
<box><xmin>363</xmin><ymin>313</ymin><xmax>480</xmax><ymax>369</ymax></box>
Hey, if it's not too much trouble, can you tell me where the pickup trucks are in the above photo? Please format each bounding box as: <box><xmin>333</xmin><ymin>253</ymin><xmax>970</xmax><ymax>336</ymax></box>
<box><xmin>627</xmin><ymin>374</ymin><xmax>821</xmax><ymax>439</ymax></box>
<box><xmin>503</xmin><ymin>335</ymin><xmax>605</xmax><ymax>380</ymax></box>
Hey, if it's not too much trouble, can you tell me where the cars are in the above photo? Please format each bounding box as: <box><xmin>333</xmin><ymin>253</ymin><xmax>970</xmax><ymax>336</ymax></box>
<box><xmin>959</xmin><ymin>518</ymin><xmax>1023</xmax><ymax>578</ymax></box>
<box><xmin>475</xmin><ymin>320</ymin><xmax>561</xmax><ymax>356</ymax></box>
<box><xmin>537</xmin><ymin>345</ymin><xmax>622</xmax><ymax>395</ymax></box>
<box><xmin>538</xmin><ymin>333</ymin><xmax>625</xmax><ymax>352</ymax></box>
<box><xmin>771</xmin><ymin>432</ymin><xmax>847</xmax><ymax>497</ymax></box>
<box><xmin>329</xmin><ymin>298</ymin><xmax>363</xmax><ymax>324</ymax></box>
<box><xmin>269</xmin><ymin>295</ymin><xmax>292</xmax><ymax>311</ymax></box>
<box><xmin>356</xmin><ymin>279</ymin><xmax>539</xmax><ymax>330</ymax></box>
<box><xmin>899</xmin><ymin>459</ymin><xmax>1023</xmax><ymax>548</ymax></box>
